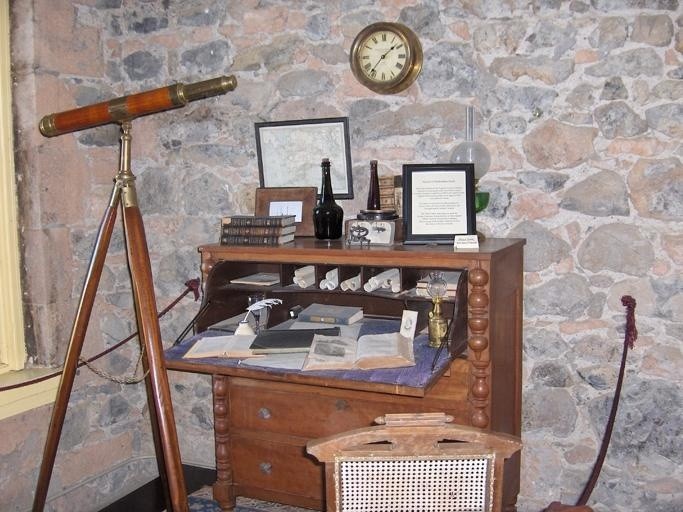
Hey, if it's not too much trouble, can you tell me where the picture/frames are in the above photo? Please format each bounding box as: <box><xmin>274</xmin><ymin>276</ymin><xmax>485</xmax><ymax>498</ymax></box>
<box><xmin>254</xmin><ymin>117</ymin><xmax>355</xmax><ymax>200</ymax></box>
<box><xmin>402</xmin><ymin>163</ymin><xmax>476</xmax><ymax>245</ymax></box>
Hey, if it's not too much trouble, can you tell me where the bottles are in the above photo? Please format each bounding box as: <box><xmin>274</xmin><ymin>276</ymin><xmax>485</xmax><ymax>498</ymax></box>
<box><xmin>312</xmin><ymin>158</ymin><xmax>343</xmax><ymax>240</ymax></box>
<box><xmin>367</xmin><ymin>160</ymin><xmax>381</xmax><ymax>211</ymax></box>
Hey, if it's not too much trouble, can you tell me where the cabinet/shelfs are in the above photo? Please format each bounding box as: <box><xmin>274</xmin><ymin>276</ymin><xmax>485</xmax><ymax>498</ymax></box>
<box><xmin>163</xmin><ymin>235</ymin><xmax>527</xmax><ymax>512</ymax></box>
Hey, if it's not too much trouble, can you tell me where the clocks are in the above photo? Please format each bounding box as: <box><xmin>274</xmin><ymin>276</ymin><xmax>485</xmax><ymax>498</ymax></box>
<box><xmin>350</xmin><ymin>22</ymin><xmax>424</xmax><ymax>95</ymax></box>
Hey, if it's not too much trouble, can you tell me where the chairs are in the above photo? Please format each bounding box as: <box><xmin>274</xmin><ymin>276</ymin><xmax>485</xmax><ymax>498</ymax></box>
<box><xmin>306</xmin><ymin>412</ymin><xmax>524</xmax><ymax>512</ymax></box>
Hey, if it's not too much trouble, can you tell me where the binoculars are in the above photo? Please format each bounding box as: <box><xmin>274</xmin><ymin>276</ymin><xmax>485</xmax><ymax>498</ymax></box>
<box><xmin>38</xmin><ymin>75</ymin><xmax>236</xmax><ymax>138</ymax></box>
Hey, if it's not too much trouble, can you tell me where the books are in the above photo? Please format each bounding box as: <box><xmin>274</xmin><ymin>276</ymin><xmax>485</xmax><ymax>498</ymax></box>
<box><xmin>249</xmin><ymin>326</ymin><xmax>340</xmax><ymax>354</ymax></box>
<box><xmin>219</xmin><ymin>215</ymin><xmax>297</xmax><ymax>246</ymax></box>
<box><xmin>416</xmin><ymin>272</ymin><xmax>461</xmax><ymax>297</ymax></box>
<box><xmin>230</xmin><ymin>271</ymin><xmax>281</xmax><ymax>287</ymax></box>
<box><xmin>298</xmin><ymin>303</ymin><xmax>364</xmax><ymax>325</ymax></box>
<box><xmin>301</xmin><ymin>331</ymin><xmax>416</xmax><ymax>372</ymax></box>
<box><xmin>183</xmin><ymin>334</ymin><xmax>265</xmax><ymax>358</ymax></box>
